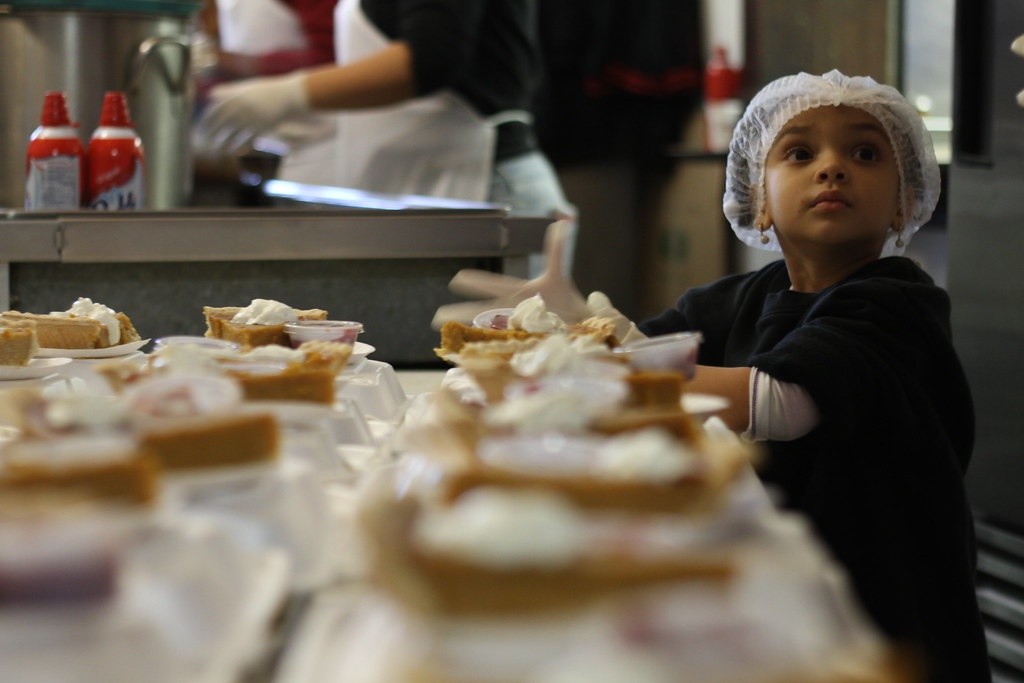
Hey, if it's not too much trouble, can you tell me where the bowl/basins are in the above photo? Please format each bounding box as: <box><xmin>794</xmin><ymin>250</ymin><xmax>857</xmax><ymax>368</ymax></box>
<box><xmin>0</xmin><ymin>294</ymin><xmax>834</xmax><ymax>682</ymax></box>
<box><xmin>471</xmin><ymin>306</ymin><xmax>565</xmax><ymax>338</ymax></box>
<box><xmin>282</xmin><ymin>321</ymin><xmax>366</xmax><ymax>351</ymax></box>
<box><xmin>612</xmin><ymin>333</ymin><xmax>704</xmax><ymax>382</ymax></box>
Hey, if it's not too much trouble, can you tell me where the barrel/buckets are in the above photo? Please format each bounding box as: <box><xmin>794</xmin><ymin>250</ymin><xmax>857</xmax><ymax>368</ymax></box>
<box><xmin>0</xmin><ymin>0</ymin><xmax>210</xmax><ymax>214</ymax></box>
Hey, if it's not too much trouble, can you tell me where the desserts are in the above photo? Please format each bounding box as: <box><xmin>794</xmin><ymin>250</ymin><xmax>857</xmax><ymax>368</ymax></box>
<box><xmin>0</xmin><ymin>298</ymin><xmax>741</xmax><ymax>615</ymax></box>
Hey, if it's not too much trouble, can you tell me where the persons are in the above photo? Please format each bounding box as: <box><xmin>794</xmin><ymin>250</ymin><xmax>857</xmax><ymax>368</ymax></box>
<box><xmin>433</xmin><ymin>71</ymin><xmax>991</xmax><ymax>683</ymax></box>
<box><xmin>182</xmin><ymin>0</ymin><xmax>563</xmax><ymax>281</ymax></box>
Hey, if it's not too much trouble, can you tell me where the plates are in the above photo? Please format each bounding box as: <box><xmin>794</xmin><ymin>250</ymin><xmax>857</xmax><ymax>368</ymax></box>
<box><xmin>1</xmin><ymin>356</ymin><xmax>72</xmax><ymax>380</ymax></box>
<box><xmin>347</xmin><ymin>341</ymin><xmax>377</xmax><ymax>366</ymax></box>
<box><xmin>677</xmin><ymin>391</ymin><xmax>732</xmax><ymax>426</ymax></box>
<box><xmin>39</xmin><ymin>337</ymin><xmax>152</xmax><ymax>359</ymax></box>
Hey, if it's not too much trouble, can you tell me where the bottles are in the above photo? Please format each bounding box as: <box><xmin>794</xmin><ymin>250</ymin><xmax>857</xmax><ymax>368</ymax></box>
<box><xmin>86</xmin><ymin>91</ymin><xmax>147</xmax><ymax>213</ymax></box>
<box><xmin>24</xmin><ymin>90</ymin><xmax>85</xmax><ymax>211</ymax></box>
